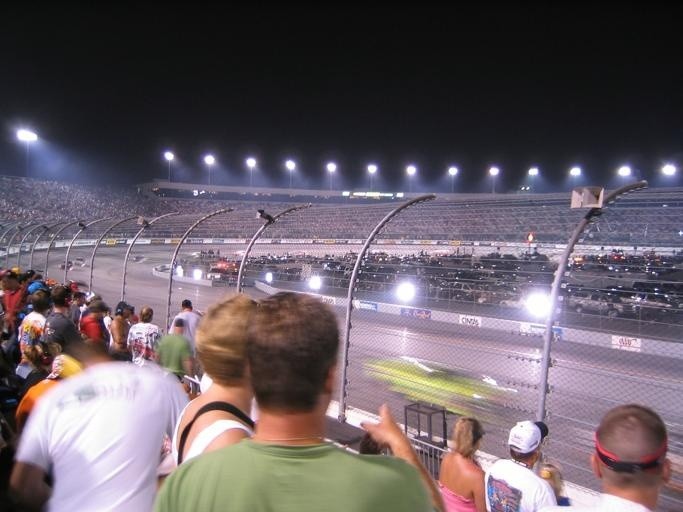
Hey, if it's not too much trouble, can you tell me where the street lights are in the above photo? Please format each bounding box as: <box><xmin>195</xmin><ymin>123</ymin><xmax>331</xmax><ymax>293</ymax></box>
<box><xmin>14</xmin><ymin>129</ymin><xmax>40</xmax><ymax>179</ymax></box>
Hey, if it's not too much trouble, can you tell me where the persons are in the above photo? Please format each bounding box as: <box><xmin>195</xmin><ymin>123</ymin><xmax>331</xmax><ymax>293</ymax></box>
<box><xmin>432</xmin><ymin>415</ymin><xmax>487</xmax><ymax>512</ymax></box>
<box><xmin>537</xmin><ymin>402</ymin><xmax>672</xmax><ymax>512</ymax></box>
<box><xmin>2</xmin><ymin>266</ymin><xmax>258</xmax><ymax>512</ymax></box>
<box><xmin>149</xmin><ymin>287</ymin><xmax>447</xmax><ymax>511</ymax></box>
<box><xmin>481</xmin><ymin>417</ymin><xmax>559</xmax><ymax>511</ymax></box>
<box><xmin>1</xmin><ymin>175</ymin><xmax>683</xmax><ymax>248</ymax></box>
<box><xmin>537</xmin><ymin>463</ymin><xmax>571</xmax><ymax>506</ymax></box>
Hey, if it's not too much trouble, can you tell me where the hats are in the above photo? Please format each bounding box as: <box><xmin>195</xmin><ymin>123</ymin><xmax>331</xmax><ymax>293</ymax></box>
<box><xmin>29</xmin><ymin>282</ymin><xmax>44</xmax><ymax>295</ymax></box>
<box><xmin>508</xmin><ymin>421</ymin><xmax>548</xmax><ymax>453</ymax></box>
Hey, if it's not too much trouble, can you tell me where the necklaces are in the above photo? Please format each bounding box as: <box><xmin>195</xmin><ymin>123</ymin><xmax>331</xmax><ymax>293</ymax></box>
<box><xmin>254</xmin><ymin>431</ymin><xmax>326</xmax><ymax>443</ymax></box>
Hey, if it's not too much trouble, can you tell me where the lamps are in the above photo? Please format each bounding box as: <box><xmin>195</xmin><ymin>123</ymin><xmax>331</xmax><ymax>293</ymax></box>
<box><xmin>255</xmin><ymin>209</ymin><xmax>273</xmax><ymax>222</ymax></box>
<box><xmin>136</xmin><ymin>217</ymin><xmax>147</xmax><ymax>228</ymax></box>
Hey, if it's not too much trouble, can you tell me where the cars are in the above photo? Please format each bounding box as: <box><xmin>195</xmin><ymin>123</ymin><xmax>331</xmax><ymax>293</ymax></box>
<box><xmin>74</xmin><ymin>256</ymin><xmax>84</xmax><ymax>265</ymax></box>
<box><xmin>361</xmin><ymin>356</ymin><xmax>515</xmax><ymax>422</ymax></box>
<box><xmin>127</xmin><ymin>254</ymin><xmax>145</xmax><ymax>263</ymax></box>
<box><xmin>61</xmin><ymin>260</ymin><xmax>71</xmax><ymax>269</ymax></box>
